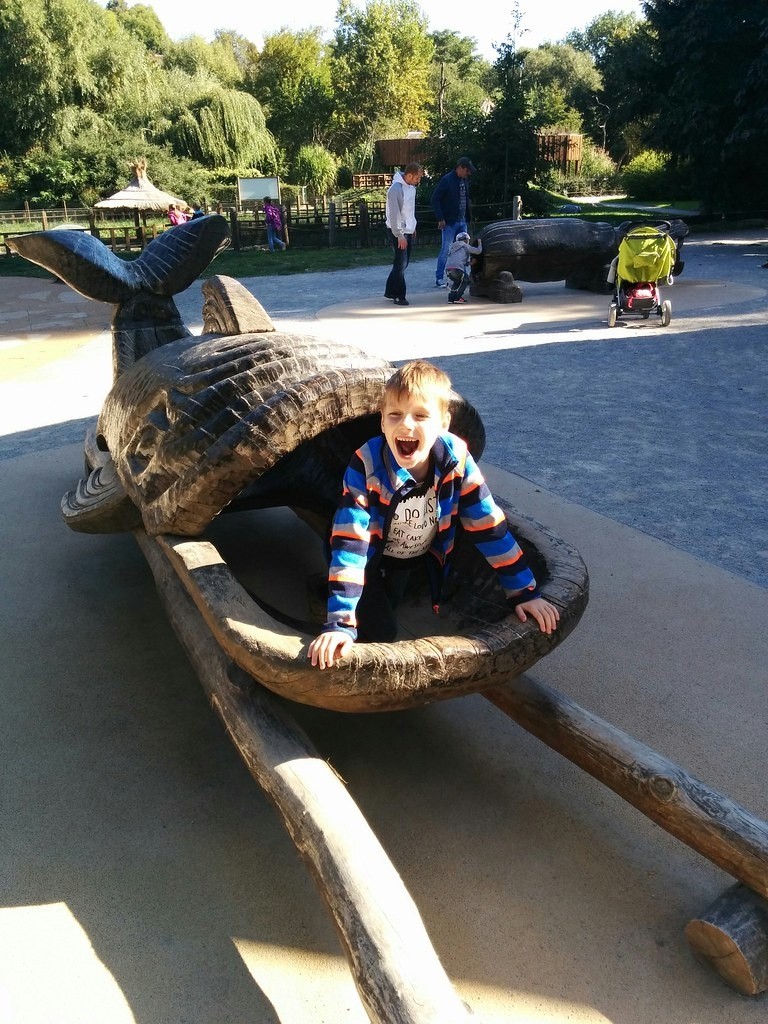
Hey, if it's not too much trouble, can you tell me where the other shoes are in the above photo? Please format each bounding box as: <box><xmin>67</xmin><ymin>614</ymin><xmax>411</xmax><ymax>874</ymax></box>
<box><xmin>282</xmin><ymin>243</ymin><xmax>286</xmax><ymax>251</ymax></box>
<box><xmin>396</xmin><ymin>603</ymin><xmax>438</xmax><ymax>638</ymax></box>
<box><xmin>309</xmin><ymin>587</ymin><xmax>330</xmax><ymax>624</ymax></box>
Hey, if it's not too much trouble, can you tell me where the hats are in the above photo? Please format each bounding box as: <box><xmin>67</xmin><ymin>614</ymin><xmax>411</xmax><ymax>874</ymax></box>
<box><xmin>456</xmin><ymin>231</ymin><xmax>471</xmax><ymax>241</ymax></box>
<box><xmin>458</xmin><ymin>157</ymin><xmax>477</xmax><ymax>172</ymax></box>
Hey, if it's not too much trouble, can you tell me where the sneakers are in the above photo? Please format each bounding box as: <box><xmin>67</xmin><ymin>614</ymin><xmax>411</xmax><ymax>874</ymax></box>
<box><xmin>384</xmin><ymin>291</ymin><xmax>394</xmax><ymax>299</ymax></box>
<box><xmin>448</xmin><ymin>296</ymin><xmax>468</xmax><ymax>304</ymax></box>
<box><xmin>436</xmin><ymin>279</ymin><xmax>446</xmax><ymax>288</ymax></box>
<box><xmin>393</xmin><ymin>296</ymin><xmax>409</xmax><ymax>305</ymax></box>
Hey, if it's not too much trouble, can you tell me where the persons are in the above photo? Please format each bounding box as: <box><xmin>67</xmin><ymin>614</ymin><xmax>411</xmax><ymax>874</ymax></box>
<box><xmin>383</xmin><ymin>163</ymin><xmax>422</xmax><ymax>305</ymax></box>
<box><xmin>446</xmin><ymin>232</ymin><xmax>482</xmax><ymax>304</ymax></box>
<box><xmin>168</xmin><ymin>204</ymin><xmax>188</xmax><ymax>226</ymax></box>
<box><xmin>191</xmin><ymin>202</ymin><xmax>204</xmax><ymax>220</ymax></box>
<box><xmin>307</xmin><ymin>360</ymin><xmax>560</xmax><ymax>670</ymax></box>
<box><xmin>263</xmin><ymin>196</ymin><xmax>286</xmax><ymax>252</ymax></box>
<box><xmin>432</xmin><ymin>158</ymin><xmax>477</xmax><ymax>288</ymax></box>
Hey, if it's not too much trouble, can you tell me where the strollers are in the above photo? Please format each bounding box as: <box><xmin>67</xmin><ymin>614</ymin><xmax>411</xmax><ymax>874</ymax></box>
<box><xmin>608</xmin><ymin>220</ymin><xmax>676</xmax><ymax>328</ymax></box>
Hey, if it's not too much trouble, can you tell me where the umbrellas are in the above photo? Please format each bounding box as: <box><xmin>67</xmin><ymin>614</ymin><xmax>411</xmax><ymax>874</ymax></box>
<box><xmin>94</xmin><ymin>161</ymin><xmax>191</xmax><ymax>226</ymax></box>
